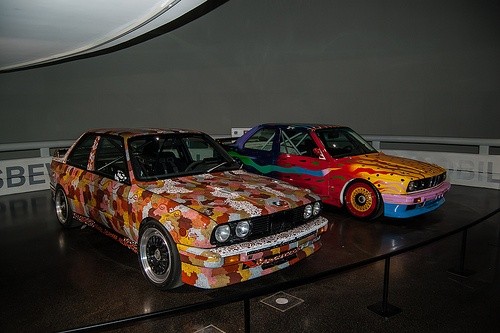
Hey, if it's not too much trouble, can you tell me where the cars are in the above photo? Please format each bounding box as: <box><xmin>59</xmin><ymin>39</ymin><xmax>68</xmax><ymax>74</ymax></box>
<box><xmin>215</xmin><ymin>120</ymin><xmax>454</xmax><ymax>222</ymax></box>
<box><xmin>49</xmin><ymin>127</ymin><xmax>330</xmax><ymax>292</ymax></box>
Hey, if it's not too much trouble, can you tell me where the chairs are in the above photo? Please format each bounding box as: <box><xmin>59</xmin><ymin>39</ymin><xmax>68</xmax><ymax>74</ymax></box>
<box><xmin>298</xmin><ymin>137</ymin><xmax>331</xmax><ymax>160</ymax></box>
<box><xmin>97</xmin><ymin>151</ymin><xmax>186</xmax><ymax>182</ymax></box>
